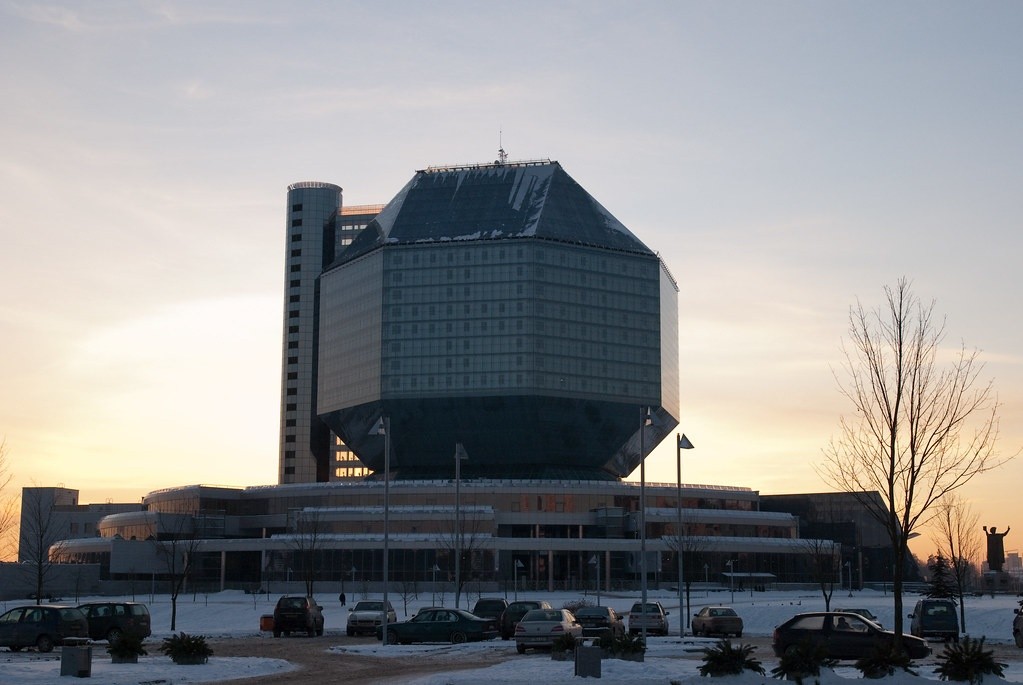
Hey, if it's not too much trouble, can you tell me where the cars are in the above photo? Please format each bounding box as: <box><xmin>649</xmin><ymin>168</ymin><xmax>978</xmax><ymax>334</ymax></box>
<box><xmin>515</xmin><ymin>609</ymin><xmax>583</xmax><ymax>654</ymax></box>
<box><xmin>692</xmin><ymin>606</ymin><xmax>744</xmax><ymax>638</ymax></box>
<box><xmin>772</xmin><ymin>612</ymin><xmax>933</xmax><ymax>664</ymax></box>
<box><xmin>377</xmin><ymin>608</ymin><xmax>499</xmax><ymax>645</ymax></box>
<box><xmin>574</xmin><ymin>606</ymin><xmax>626</xmax><ymax>639</ymax></box>
<box><xmin>833</xmin><ymin>607</ymin><xmax>882</xmax><ymax>632</ymax></box>
<box><xmin>500</xmin><ymin>600</ymin><xmax>552</xmax><ymax>641</ymax></box>
<box><xmin>472</xmin><ymin>597</ymin><xmax>509</xmax><ymax>626</ymax></box>
<box><xmin>1012</xmin><ymin>606</ymin><xmax>1023</xmax><ymax>649</ymax></box>
<box><xmin>412</xmin><ymin>605</ymin><xmax>446</xmax><ymax>621</ymax></box>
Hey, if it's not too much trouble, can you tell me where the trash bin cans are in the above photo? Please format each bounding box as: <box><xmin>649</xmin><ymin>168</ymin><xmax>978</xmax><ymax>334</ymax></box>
<box><xmin>59</xmin><ymin>636</ymin><xmax>93</xmax><ymax>678</ymax></box>
<box><xmin>572</xmin><ymin>636</ymin><xmax>602</xmax><ymax>679</ymax></box>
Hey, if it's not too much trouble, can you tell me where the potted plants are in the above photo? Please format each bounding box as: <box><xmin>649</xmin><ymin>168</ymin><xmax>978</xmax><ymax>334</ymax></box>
<box><xmin>105</xmin><ymin>628</ymin><xmax>148</xmax><ymax>664</ymax></box>
<box><xmin>157</xmin><ymin>631</ymin><xmax>214</xmax><ymax>665</ymax></box>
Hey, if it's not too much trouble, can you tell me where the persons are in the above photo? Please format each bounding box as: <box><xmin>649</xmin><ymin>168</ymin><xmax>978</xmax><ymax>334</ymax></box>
<box><xmin>738</xmin><ymin>581</ymin><xmax>746</xmax><ymax>592</ymax></box>
<box><xmin>339</xmin><ymin>592</ymin><xmax>346</xmax><ymax>607</ymax></box>
<box><xmin>983</xmin><ymin>525</ymin><xmax>1010</xmax><ymax>572</ymax></box>
<box><xmin>837</xmin><ymin>617</ymin><xmax>851</xmax><ymax>630</ymax></box>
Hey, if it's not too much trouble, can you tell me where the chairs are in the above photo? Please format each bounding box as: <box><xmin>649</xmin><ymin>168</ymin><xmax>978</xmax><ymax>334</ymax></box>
<box><xmin>94</xmin><ymin>608</ymin><xmax>110</xmax><ymax>617</ymax></box>
<box><xmin>25</xmin><ymin>614</ymin><xmax>34</xmax><ymax>622</ymax></box>
<box><xmin>836</xmin><ymin>617</ymin><xmax>853</xmax><ymax>629</ymax></box>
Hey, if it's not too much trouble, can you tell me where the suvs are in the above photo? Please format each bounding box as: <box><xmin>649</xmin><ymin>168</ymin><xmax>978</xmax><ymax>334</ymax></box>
<box><xmin>272</xmin><ymin>595</ymin><xmax>324</xmax><ymax>637</ymax></box>
<box><xmin>907</xmin><ymin>597</ymin><xmax>960</xmax><ymax>643</ymax></box>
<box><xmin>79</xmin><ymin>602</ymin><xmax>151</xmax><ymax>645</ymax></box>
<box><xmin>347</xmin><ymin>599</ymin><xmax>397</xmax><ymax>637</ymax></box>
<box><xmin>629</xmin><ymin>601</ymin><xmax>671</xmax><ymax>636</ymax></box>
<box><xmin>0</xmin><ymin>605</ymin><xmax>93</xmax><ymax>654</ymax></box>
